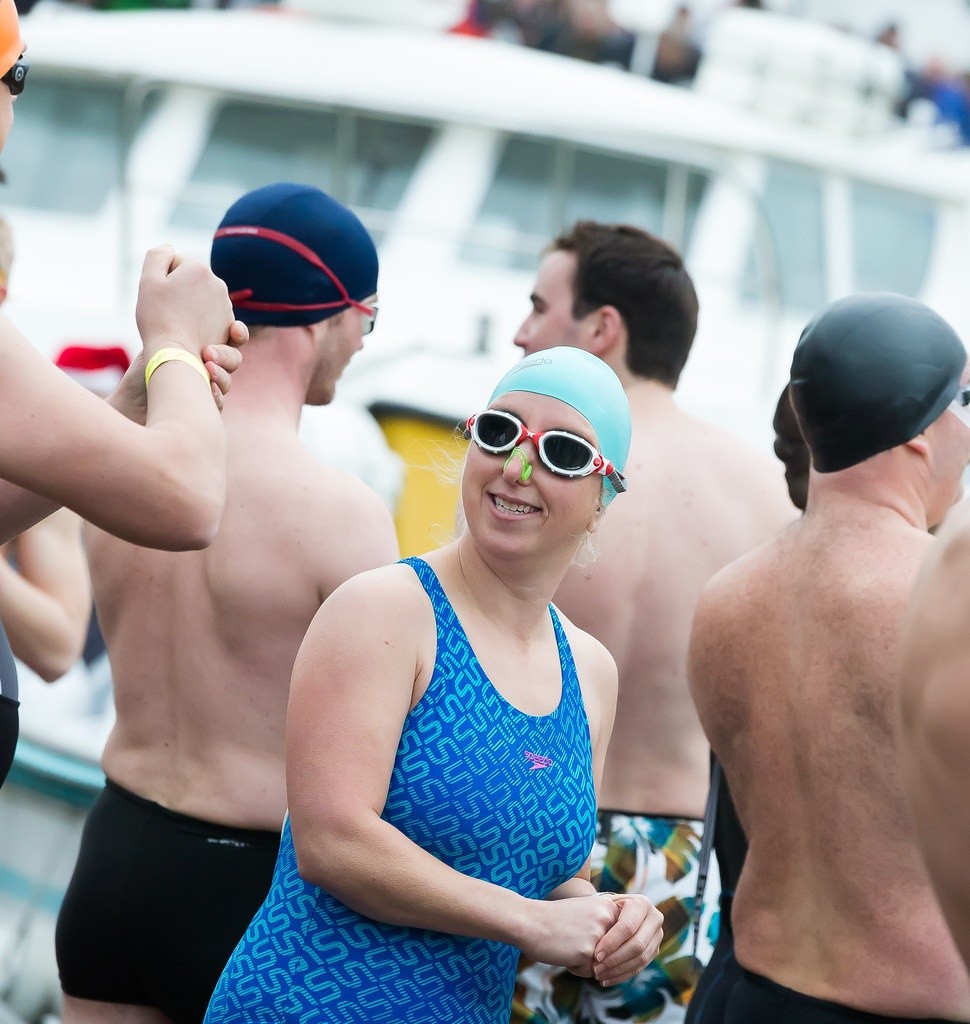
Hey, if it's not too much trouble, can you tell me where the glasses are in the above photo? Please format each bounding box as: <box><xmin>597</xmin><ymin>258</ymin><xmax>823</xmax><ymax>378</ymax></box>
<box><xmin>0</xmin><ymin>65</ymin><xmax>29</xmax><ymax>95</ymax></box>
<box><xmin>464</xmin><ymin>409</ymin><xmax>614</xmax><ymax>480</ymax></box>
<box><xmin>349</xmin><ymin>301</ymin><xmax>377</xmax><ymax>336</ymax></box>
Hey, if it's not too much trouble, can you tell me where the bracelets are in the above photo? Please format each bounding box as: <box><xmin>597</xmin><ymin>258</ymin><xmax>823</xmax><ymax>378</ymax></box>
<box><xmin>144</xmin><ymin>346</ymin><xmax>214</xmax><ymax>396</ymax></box>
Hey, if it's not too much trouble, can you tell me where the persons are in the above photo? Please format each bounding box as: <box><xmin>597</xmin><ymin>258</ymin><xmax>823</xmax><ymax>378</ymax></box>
<box><xmin>55</xmin><ymin>177</ymin><xmax>403</xmax><ymax>1024</ymax></box>
<box><xmin>0</xmin><ymin>207</ymin><xmax>93</xmax><ymax>784</ymax></box>
<box><xmin>199</xmin><ymin>345</ymin><xmax>666</xmax><ymax>1024</ymax></box>
<box><xmin>506</xmin><ymin>221</ymin><xmax>790</xmax><ymax>1023</ymax></box>
<box><xmin>673</xmin><ymin>291</ymin><xmax>969</xmax><ymax>1024</ymax></box>
<box><xmin>449</xmin><ymin>0</ymin><xmax>970</xmax><ymax>144</ymax></box>
<box><xmin>0</xmin><ymin>0</ymin><xmax>248</xmax><ymax>551</ymax></box>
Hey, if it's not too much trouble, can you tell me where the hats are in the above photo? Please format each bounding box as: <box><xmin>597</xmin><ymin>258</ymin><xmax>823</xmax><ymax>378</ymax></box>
<box><xmin>210</xmin><ymin>182</ymin><xmax>378</xmax><ymax>325</ymax></box>
<box><xmin>0</xmin><ymin>0</ymin><xmax>26</xmax><ymax>79</ymax></box>
<box><xmin>787</xmin><ymin>292</ymin><xmax>968</xmax><ymax>473</ymax></box>
<box><xmin>771</xmin><ymin>385</ymin><xmax>809</xmax><ymax>508</ymax></box>
<box><xmin>485</xmin><ymin>345</ymin><xmax>632</xmax><ymax>509</ymax></box>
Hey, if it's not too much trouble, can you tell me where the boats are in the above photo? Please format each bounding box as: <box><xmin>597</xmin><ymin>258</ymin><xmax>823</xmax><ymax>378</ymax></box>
<box><xmin>3</xmin><ymin>0</ymin><xmax>970</xmax><ymax>1024</ymax></box>
